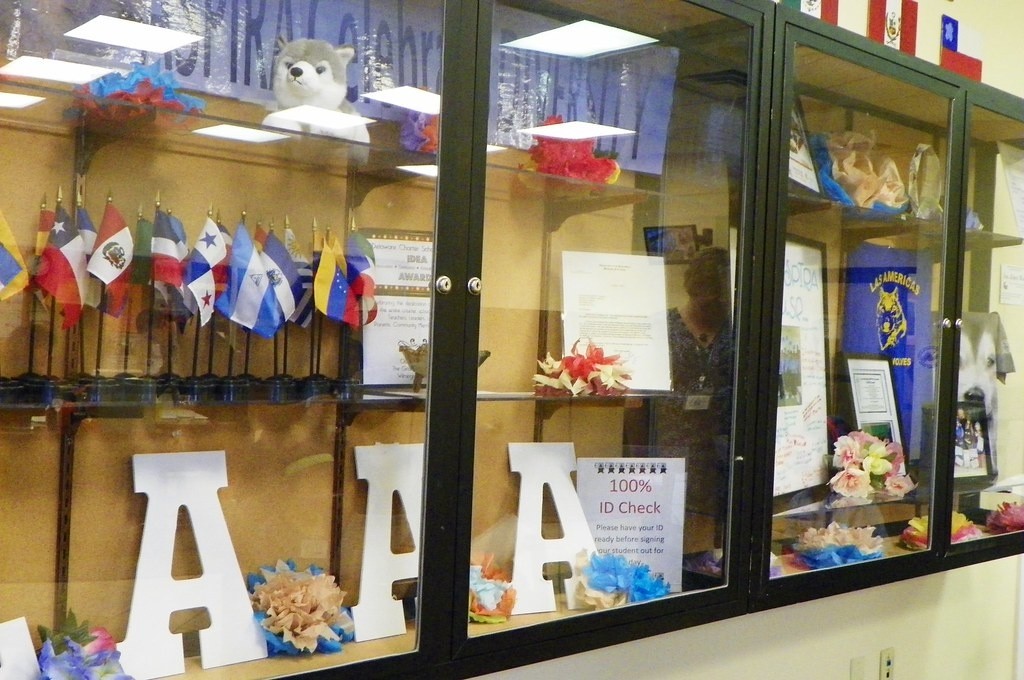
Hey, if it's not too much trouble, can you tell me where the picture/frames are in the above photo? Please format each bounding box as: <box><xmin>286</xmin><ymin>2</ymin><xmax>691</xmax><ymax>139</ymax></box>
<box><xmin>954</xmin><ymin>399</ymin><xmax>994</xmax><ymax>486</ymax></box>
<box><xmin>837</xmin><ymin>351</ymin><xmax>911</xmax><ymax>478</ymax></box>
<box><xmin>715</xmin><ymin>217</ymin><xmax>835</xmax><ymax>514</ymax></box>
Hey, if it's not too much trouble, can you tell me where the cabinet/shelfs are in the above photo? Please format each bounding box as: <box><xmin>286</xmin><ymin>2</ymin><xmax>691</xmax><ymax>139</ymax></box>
<box><xmin>0</xmin><ymin>1</ymin><xmax>1022</xmax><ymax>675</ymax></box>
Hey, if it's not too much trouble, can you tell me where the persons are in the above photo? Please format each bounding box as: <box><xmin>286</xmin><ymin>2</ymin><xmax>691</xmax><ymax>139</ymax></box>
<box><xmin>622</xmin><ymin>247</ymin><xmax>735</xmax><ymax>553</ymax></box>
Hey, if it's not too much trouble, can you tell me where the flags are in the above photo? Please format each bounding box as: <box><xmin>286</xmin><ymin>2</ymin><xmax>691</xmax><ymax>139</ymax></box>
<box><xmin>0</xmin><ymin>202</ymin><xmax>379</xmax><ymax>353</ymax></box>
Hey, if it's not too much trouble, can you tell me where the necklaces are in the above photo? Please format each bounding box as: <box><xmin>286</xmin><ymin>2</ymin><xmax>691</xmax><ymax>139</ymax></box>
<box><xmin>694</xmin><ymin>331</ymin><xmax>713</xmax><ymax>341</ymax></box>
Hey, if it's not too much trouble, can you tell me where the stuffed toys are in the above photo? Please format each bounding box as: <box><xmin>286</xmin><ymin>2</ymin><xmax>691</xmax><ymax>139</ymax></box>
<box><xmin>272</xmin><ymin>34</ymin><xmax>373</xmax><ymax>163</ymax></box>
